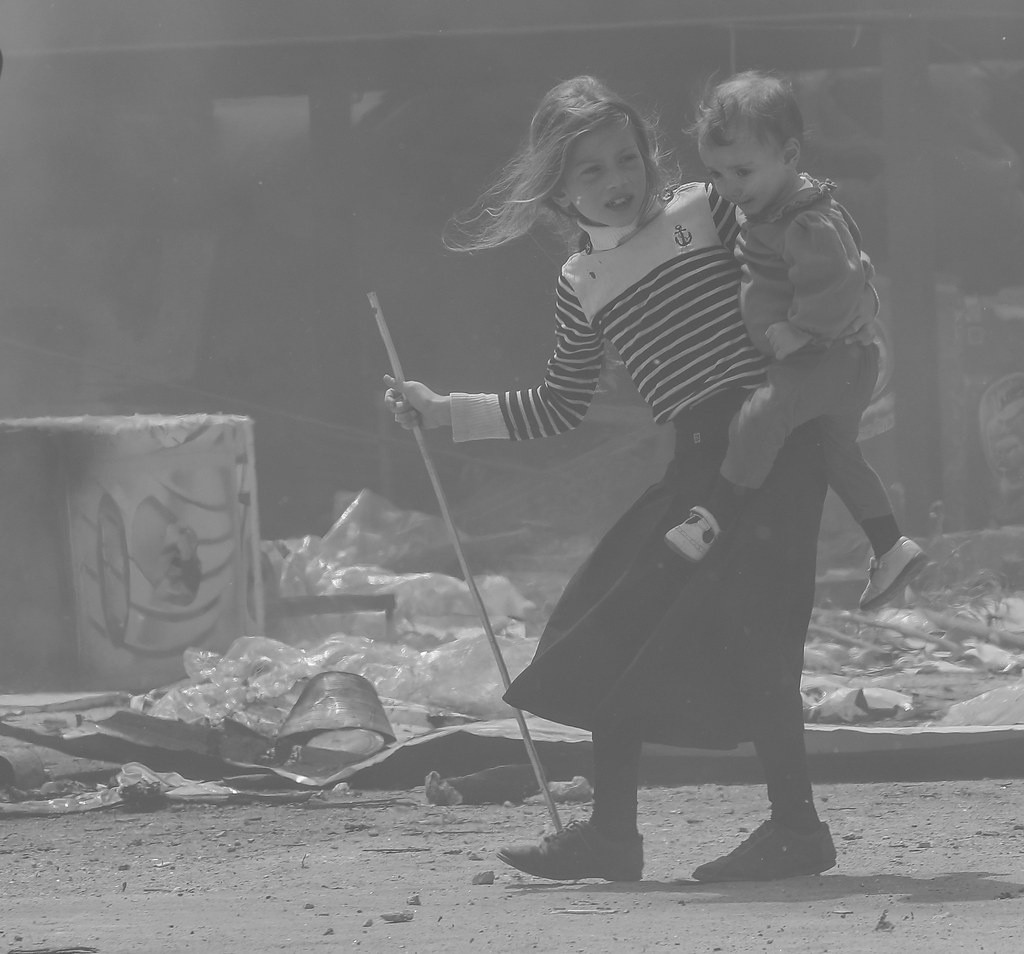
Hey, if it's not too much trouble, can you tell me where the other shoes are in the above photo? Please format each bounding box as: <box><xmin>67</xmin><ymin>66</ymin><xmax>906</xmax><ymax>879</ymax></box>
<box><xmin>692</xmin><ymin>817</ymin><xmax>837</xmax><ymax>882</ymax></box>
<box><xmin>497</xmin><ymin>819</ymin><xmax>644</xmax><ymax>882</ymax></box>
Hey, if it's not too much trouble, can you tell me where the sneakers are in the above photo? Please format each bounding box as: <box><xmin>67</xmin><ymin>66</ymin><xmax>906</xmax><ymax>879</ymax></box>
<box><xmin>663</xmin><ymin>504</ymin><xmax>723</xmax><ymax>566</ymax></box>
<box><xmin>859</xmin><ymin>535</ymin><xmax>929</xmax><ymax>611</ymax></box>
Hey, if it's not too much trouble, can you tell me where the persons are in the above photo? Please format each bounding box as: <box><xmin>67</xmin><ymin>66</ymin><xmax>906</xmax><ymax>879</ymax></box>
<box><xmin>664</xmin><ymin>73</ymin><xmax>929</xmax><ymax>610</ymax></box>
<box><xmin>381</xmin><ymin>73</ymin><xmax>843</xmax><ymax>884</ymax></box>
